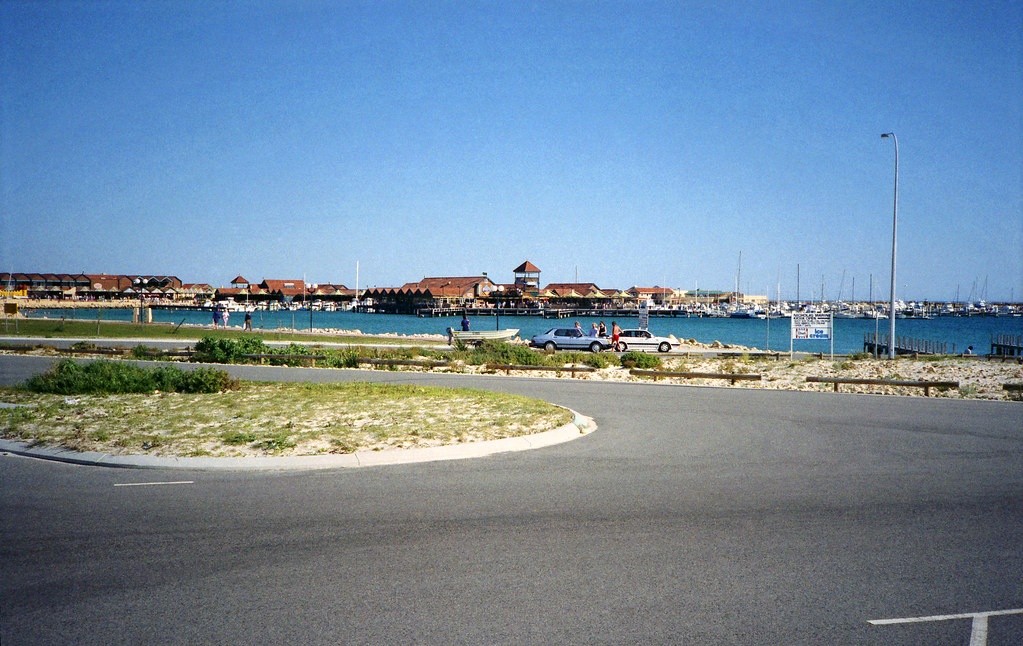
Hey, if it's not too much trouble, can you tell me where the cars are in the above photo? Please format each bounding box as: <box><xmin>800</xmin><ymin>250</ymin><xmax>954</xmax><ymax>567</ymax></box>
<box><xmin>606</xmin><ymin>329</ymin><xmax>681</xmax><ymax>352</ymax></box>
<box><xmin>531</xmin><ymin>328</ymin><xmax>612</xmax><ymax>354</ymax></box>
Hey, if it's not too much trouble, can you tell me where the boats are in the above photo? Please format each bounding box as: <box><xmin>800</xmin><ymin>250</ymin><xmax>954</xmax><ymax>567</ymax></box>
<box><xmin>202</xmin><ymin>297</ymin><xmax>378</xmax><ymax>312</ymax></box>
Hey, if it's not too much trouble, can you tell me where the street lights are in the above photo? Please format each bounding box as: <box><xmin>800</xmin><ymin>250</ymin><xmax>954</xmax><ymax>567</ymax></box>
<box><xmin>881</xmin><ymin>132</ymin><xmax>899</xmax><ymax>359</ymax></box>
<box><xmin>134</xmin><ymin>276</ymin><xmax>148</xmax><ymax>323</ymax></box>
<box><xmin>306</xmin><ymin>283</ymin><xmax>318</xmax><ymax>332</ymax></box>
<box><xmin>491</xmin><ymin>285</ymin><xmax>504</xmax><ymax>330</ymax></box>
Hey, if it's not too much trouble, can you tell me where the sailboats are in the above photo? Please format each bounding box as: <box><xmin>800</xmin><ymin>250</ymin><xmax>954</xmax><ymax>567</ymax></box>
<box><xmin>350</xmin><ymin>260</ymin><xmax>361</xmax><ymax>311</ymax></box>
<box><xmin>654</xmin><ymin>251</ymin><xmax>1023</xmax><ymax>320</ymax></box>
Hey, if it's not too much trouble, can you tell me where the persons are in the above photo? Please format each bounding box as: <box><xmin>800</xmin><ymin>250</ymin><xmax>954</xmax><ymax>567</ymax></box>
<box><xmin>612</xmin><ymin>321</ymin><xmax>624</xmax><ymax>352</ymax></box>
<box><xmin>599</xmin><ymin>321</ymin><xmax>607</xmax><ymax>338</ymax></box>
<box><xmin>222</xmin><ymin>309</ymin><xmax>229</xmax><ymax>328</ymax></box>
<box><xmin>244</xmin><ymin>311</ymin><xmax>252</xmax><ymax>332</ymax></box>
<box><xmin>574</xmin><ymin>321</ymin><xmax>583</xmax><ymax>336</ymax></box>
<box><xmin>461</xmin><ymin>316</ymin><xmax>470</xmax><ymax>331</ymax></box>
<box><xmin>212</xmin><ymin>309</ymin><xmax>220</xmax><ymax>329</ymax></box>
<box><xmin>964</xmin><ymin>346</ymin><xmax>973</xmax><ymax>354</ymax></box>
<box><xmin>588</xmin><ymin>323</ymin><xmax>599</xmax><ymax>338</ymax></box>
